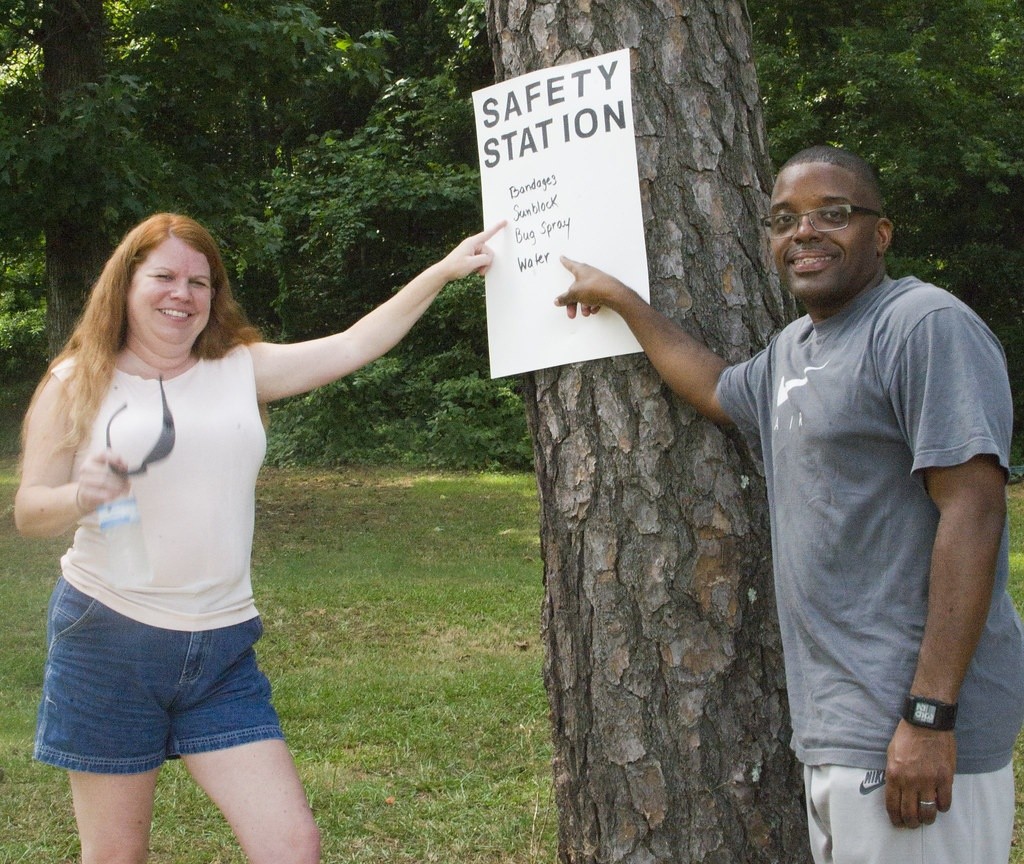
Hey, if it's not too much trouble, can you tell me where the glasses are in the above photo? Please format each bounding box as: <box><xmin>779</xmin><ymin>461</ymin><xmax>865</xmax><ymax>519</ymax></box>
<box><xmin>760</xmin><ymin>204</ymin><xmax>883</xmax><ymax>239</ymax></box>
<box><xmin>106</xmin><ymin>374</ymin><xmax>175</xmax><ymax>478</ymax></box>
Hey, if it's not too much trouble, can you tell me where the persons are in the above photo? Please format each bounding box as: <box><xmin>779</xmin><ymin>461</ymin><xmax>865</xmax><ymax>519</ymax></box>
<box><xmin>554</xmin><ymin>146</ymin><xmax>1024</xmax><ymax>864</ymax></box>
<box><xmin>12</xmin><ymin>210</ymin><xmax>508</xmax><ymax>864</ymax></box>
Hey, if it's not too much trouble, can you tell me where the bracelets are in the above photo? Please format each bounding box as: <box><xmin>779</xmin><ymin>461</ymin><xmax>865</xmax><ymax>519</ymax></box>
<box><xmin>76</xmin><ymin>487</ymin><xmax>97</xmax><ymax>513</ymax></box>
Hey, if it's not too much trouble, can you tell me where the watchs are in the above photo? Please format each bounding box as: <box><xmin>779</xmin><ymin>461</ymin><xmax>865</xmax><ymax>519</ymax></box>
<box><xmin>900</xmin><ymin>695</ymin><xmax>959</xmax><ymax>731</ymax></box>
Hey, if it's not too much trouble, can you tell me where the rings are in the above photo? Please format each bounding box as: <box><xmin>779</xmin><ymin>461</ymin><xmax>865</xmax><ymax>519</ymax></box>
<box><xmin>920</xmin><ymin>801</ymin><xmax>935</xmax><ymax>805</ymax></box>
